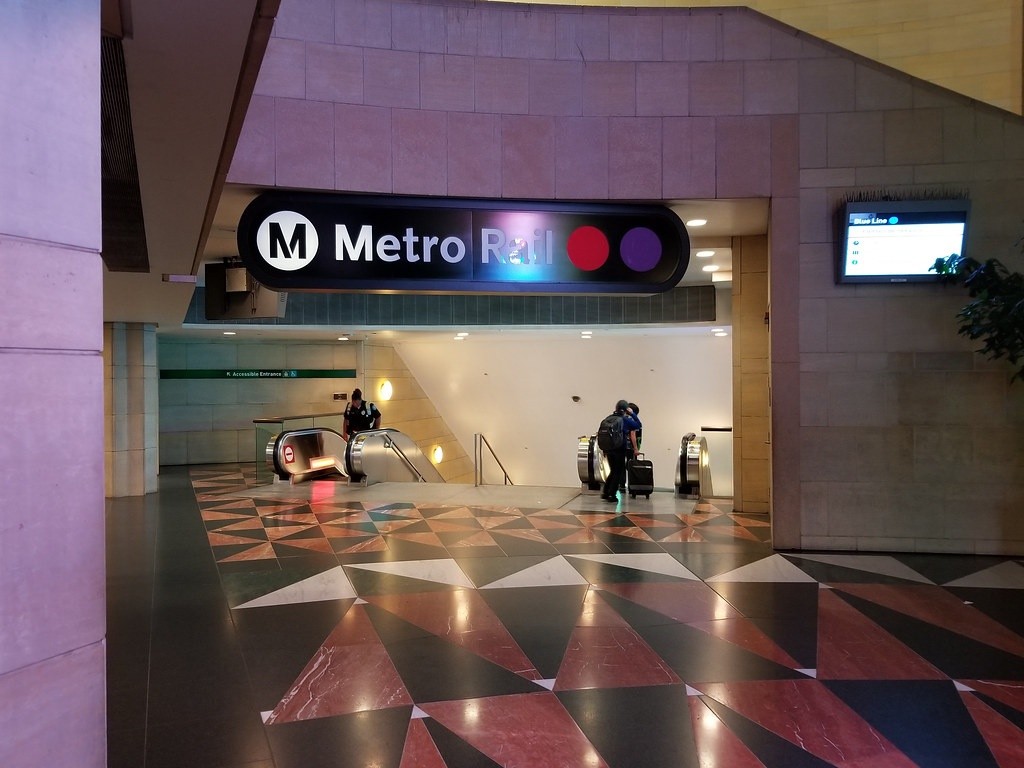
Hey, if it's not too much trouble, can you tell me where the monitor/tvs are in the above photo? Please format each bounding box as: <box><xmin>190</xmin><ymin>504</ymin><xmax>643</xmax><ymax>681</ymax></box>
<box><xmin>838</xmin><ymin>199</ymin><xmax>971</xmax><ymax>281</ymax></box>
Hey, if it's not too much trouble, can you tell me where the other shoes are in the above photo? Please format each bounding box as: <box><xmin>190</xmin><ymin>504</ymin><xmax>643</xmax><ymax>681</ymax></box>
<box><xmin>601</xmin><ymin>493</ymin><xmax>618</xmax><ymax>502</ymax></box>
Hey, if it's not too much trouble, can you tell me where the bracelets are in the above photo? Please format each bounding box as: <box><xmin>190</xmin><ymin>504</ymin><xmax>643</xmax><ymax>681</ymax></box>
<box><xmin>633</xmin><ymin>448</ymin><xmax>638</xmax><ymax>450</ymax></box>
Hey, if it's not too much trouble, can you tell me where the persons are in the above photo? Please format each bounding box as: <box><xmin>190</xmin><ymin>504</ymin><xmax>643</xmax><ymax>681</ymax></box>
<box><xmin>342</xmin><ymin>388</ymin><xmax>381</xmax><ymax>442</ymax></box>
<box><xmin>598</xmin><ymin>400</ymin><xmax>642</xmax><ymax>502</ymax></box>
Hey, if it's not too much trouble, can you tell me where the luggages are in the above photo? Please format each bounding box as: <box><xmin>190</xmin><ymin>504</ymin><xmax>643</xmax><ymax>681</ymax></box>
<box><xmin>627</xmin><ymin>453</ymin><xmax>654</xmax><ymax>499</ymax></box>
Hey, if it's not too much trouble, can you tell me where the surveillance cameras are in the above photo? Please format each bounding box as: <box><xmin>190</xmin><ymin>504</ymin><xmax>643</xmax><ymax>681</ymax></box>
<box><xmin>572</xmin><ymin>396</ymin><xmax>580</xmax><ymax>402</ymax></box>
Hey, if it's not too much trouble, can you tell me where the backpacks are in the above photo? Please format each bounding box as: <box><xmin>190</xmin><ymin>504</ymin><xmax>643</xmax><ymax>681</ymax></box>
<box><xmin>597</xmin><ymin>415</ymin><xmax>629</xmax><ymax>454</ymax></box>
